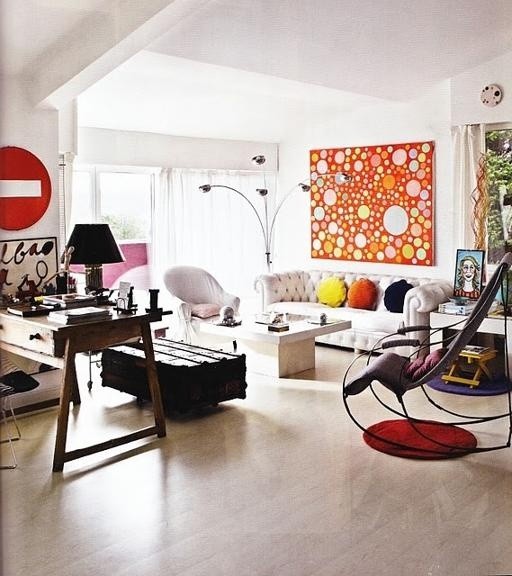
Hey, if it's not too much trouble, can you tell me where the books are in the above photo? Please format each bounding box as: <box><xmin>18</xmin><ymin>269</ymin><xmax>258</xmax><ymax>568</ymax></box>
<box><xmin>43</xmin><ymin>294</ymin><xmax>112</xmax><ymax>325</ymax></box>
<box><xmin>461</xmin><ymin>345</ymin><xmax>489</xmax><ymax>354</ymax></box>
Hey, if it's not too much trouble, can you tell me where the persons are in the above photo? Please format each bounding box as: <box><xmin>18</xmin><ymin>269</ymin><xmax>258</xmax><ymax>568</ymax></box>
<box><xmin>454</xmin><ymin>256</ymin><xmax>480</xmax><ymax>299</ymax></box>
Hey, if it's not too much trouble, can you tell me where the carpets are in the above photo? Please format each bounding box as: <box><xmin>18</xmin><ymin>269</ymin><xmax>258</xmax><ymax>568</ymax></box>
<box><xmin>426</xmin><ymin>373</ymin><xmax>511</xmax><ymax>397</ymax></box>
<box><xmin>362</xmin><ymin>419</ymin><xmax>478</xmax><ymax>461</ymax></box>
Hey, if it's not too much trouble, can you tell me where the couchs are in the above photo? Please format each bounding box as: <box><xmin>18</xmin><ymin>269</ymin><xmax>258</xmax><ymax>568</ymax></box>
<box><xmin>253</xmin><ymin>269</ymin><xmax>453</xmax><ymax>363</ymax></box>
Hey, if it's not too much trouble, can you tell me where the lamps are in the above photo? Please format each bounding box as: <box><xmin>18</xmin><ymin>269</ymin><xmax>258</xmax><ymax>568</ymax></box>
<box><xmin>59</xmin><ymin>223</ymin><xmax>128</xmax><ymax>295</ymax></box>
<box><xmin>198</xmin><ymin>154</ymin><xmax>352</xmax><ymax>270</ymax></box>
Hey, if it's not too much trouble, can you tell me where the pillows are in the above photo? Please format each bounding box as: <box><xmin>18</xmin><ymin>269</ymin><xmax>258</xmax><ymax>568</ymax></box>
<box><xmin>347</xmin><ymin>277</ymin><xmax>379</xmax><ymax>312</ymax></box>
<box><xmin>406</xmin><ymin>352</ymin><xmax>425</xmax><ymax>375</ymax></box>
<box><xmin>316</xmin><ymin>275</ymin><xmax>347</xmax><ymax>309</ymax></box>
<box><xmin>383</xmin><ymin>278</ymin><xmax>414</xmax><ymax>314</ymax></box>
<box><xmin>190</xmin><ymin>302</ymin><xmax>220</xmax><ymax>317</ymax></box>
<box><xmin>411</xmin><ymin>346</ymin><xmax>447</xmax><ymax>382</ymax></box>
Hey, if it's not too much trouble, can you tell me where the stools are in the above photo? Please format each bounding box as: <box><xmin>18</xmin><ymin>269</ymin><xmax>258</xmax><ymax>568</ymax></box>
<box><xmin>0</xmin><ymin>351</ymin><xmax>40</xmax><ymax>470</ymax></box>
<box><xmin>440</xmin><ymin>344</ymin><xmax>497</xmax><ymax>390</ymax></box>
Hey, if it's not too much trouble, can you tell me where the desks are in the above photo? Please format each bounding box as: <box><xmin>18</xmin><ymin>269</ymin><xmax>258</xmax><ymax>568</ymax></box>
<box><xmin>428</xmin><ymin>309</ymin><xmax>512</xmax><ymax>383</ymax></box>
<box><xmin>100</xmin><ymin>338</ymin><xmax>249</xmax><ymax>423</ymax></box>
<box><xmin>0</xmin><ymin>304</ymin><xmax>174</xmax><ymax>473</ymax></box>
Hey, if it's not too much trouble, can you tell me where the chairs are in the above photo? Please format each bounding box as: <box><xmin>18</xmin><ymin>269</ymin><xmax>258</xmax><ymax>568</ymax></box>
<box><xmin>164</xmin><ymin>265</ymin><xmax>241</xmax><ymax>344</ymax></box>
<box><xmin>341</xmin><ymin>250</ymin><xmax>512</xmax><ymax>460</ymax></box>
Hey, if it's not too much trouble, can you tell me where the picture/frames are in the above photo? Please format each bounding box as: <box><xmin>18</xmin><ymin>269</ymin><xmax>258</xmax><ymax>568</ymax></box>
<box><xmin>453</xmin><ymin>248</ymin><xmax>485</xmax><ymax>302</ymax></box>
<box><xmin>1</xmin><ymin>236</ymin><xmax>60</xmax><ymax>299</ymax></box>
